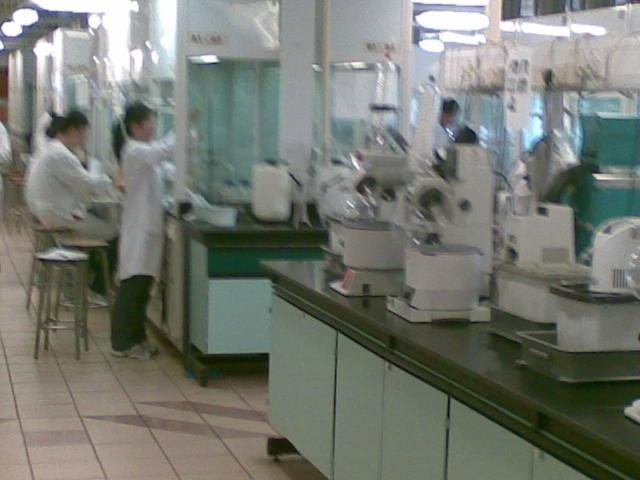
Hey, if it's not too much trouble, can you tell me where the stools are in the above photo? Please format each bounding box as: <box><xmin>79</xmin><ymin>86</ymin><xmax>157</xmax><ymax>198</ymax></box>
<box><xmin>3</xmin><ymin>169</ymin><xmax>112</xmax><ymax>360</ymax></box>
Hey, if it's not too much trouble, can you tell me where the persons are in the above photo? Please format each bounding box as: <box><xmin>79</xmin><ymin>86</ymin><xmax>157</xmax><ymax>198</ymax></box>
<box><xmin>23</xmin><ymin>110</ymin><xmax>126</xmax><ymax>308</ymax></box>
<box><xmin>111</xmin><ymin>101</ymin><xmax>203</xmax><ymax>360</ymax></box>
<box><xmin>434</xmin><ymin>99</ymin><xmax>459</xmax><ymax>148</ymax></box>
<box><xmin>0</xmin><ymin>121</ymin><xmax>12</xmax><ymax>201</ymax></box>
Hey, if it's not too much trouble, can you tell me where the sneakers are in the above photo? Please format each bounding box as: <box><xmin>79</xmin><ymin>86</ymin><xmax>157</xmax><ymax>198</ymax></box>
<box><xmin>111</xmin><ymin>340</ymin><xmax>158</xmax><ymax>359</ymax></box>
